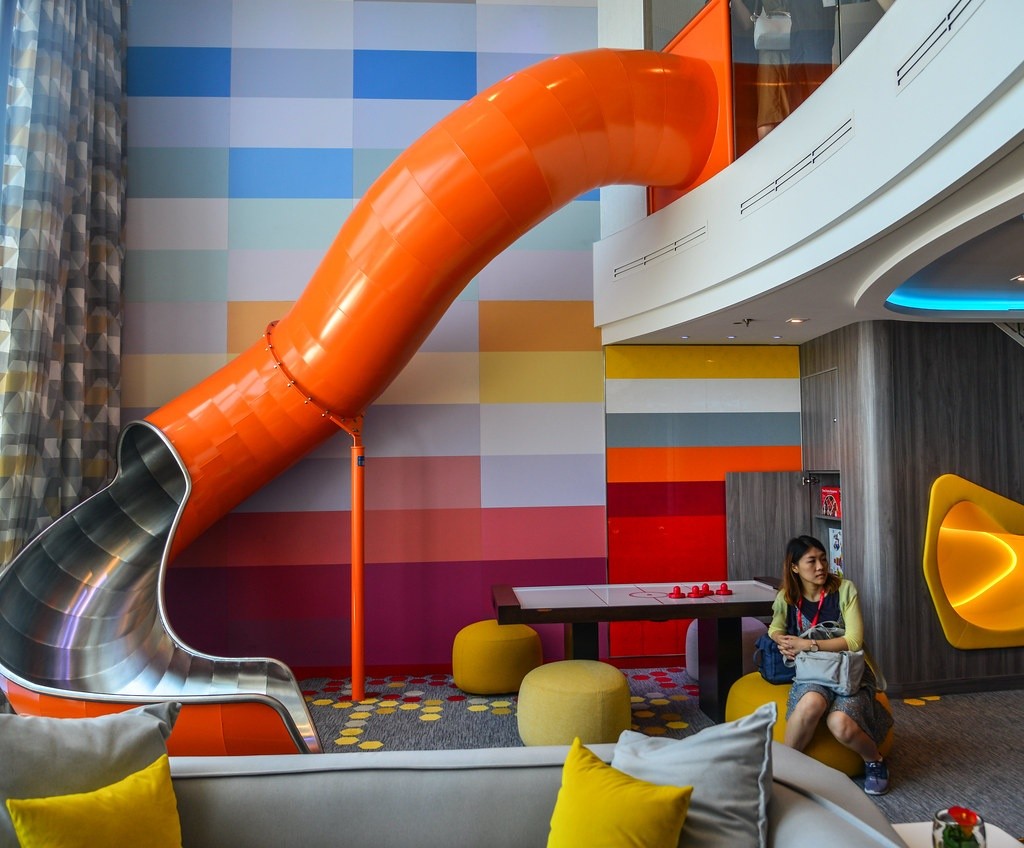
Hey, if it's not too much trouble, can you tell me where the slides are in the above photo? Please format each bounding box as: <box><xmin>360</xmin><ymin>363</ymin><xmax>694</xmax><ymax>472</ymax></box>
<box><xmin>0</xmin><ymin>45</ymin><xmax>720</xmax><ymax>760</ymax></box>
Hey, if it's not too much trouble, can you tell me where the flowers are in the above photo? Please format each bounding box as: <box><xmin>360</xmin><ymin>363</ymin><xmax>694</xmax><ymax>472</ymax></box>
<box><xmin>949</xmin><ymin>805</ymin><xmax>976</xmax><ymax>837</ymax></box>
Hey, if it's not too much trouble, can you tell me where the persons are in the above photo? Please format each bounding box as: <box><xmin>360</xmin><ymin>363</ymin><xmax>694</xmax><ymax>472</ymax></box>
<box><xmin>752</xmin><ymin>0</ymin><xmax>837</xmax><ymax>141</ymax></box>
<box><xmin>767</xmin><ymin>534</ymin><xmax>895</xmax><ymax>795</ymax></box>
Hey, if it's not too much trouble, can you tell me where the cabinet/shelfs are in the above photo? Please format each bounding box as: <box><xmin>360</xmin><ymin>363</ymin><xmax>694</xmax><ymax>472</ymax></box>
<box><xmin>724</xmin><ymin>320</ymin><xmax>1024</xmax><ymax>702</ymax></box>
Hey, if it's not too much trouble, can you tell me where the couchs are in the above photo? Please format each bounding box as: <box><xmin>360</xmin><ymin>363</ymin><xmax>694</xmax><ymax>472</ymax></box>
<box><xmin>167</xmin><ymin>741</ymin><xmax>910</xmax><ymax>848</ymax></box>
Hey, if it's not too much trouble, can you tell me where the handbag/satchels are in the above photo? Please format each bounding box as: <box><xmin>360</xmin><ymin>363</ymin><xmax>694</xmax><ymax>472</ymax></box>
<box><xmin>783</xmin><ymin>621</ymin><xmax>864</xmax><ymax>696</ymax></box>
<box><xmin>752</xmin><ymin>602</ymin><xmax>795</xmax><ymax>685</ymax></box>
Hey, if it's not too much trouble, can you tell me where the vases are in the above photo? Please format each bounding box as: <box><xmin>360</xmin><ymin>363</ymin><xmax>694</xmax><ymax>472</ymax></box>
<box><xmin>931</xmin><ymin>809</ymin><xmax>986</xmax><ymax>848</ymax></box>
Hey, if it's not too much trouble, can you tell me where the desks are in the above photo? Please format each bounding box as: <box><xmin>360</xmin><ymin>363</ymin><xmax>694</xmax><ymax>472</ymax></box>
<box><xmin>490</xmin><ymin>579</ymin><xmax>780</xmax><ymax>719</ymax></box>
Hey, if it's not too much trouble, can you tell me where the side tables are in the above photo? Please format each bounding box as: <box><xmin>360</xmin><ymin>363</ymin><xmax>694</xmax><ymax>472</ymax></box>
<box><xmin>891</xmin><ymin>820</ymin><xmax>1024</xmax><ymax>848</ymax></box>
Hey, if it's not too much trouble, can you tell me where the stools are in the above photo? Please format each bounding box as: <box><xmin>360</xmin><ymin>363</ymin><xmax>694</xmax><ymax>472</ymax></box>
<box><xmin>517</xmin><ymin>660</ymin><xmax>631</xmax><ymax>747</ymax></box>
<box><xmin>685</xmin><ymin>617</ymin><xmax>767</xmax><ymax>681</ymax></box>
<box><xmin>725</xmin><ymin>671</ymin><xmax>895</xmax><ymax>778</ymax></box>
<box><xmin>452</xmin><ymin>621</ymin><xmax>543</xmax><ymax>695</ymax></box>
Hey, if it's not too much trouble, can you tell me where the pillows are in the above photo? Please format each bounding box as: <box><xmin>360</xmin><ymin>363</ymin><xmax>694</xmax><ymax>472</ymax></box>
<box><xmin>7</xmin><ymin>754</ymin><xmax>185</xmax><ymax>848</ymax></box>
<box><xmin>611</xmin><ymin>702</ymin><xmax>778</xmax><ymax>848</ymax></box>
<box><xmin>0</xmin><ymin>701</ymin><xmax>181</xmax><ymax>848</ymax></box>
<box><xmin>547</xmin><ymin>738</ymin><xmax>694</xmax><ymax>848</ymax></box>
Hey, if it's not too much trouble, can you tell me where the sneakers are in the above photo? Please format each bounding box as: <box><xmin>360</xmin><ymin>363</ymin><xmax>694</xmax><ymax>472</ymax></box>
<box><xmin>864</xmin><ymin>752</ymin><xmax>890</xmax><ymax>795</ymax></box>
